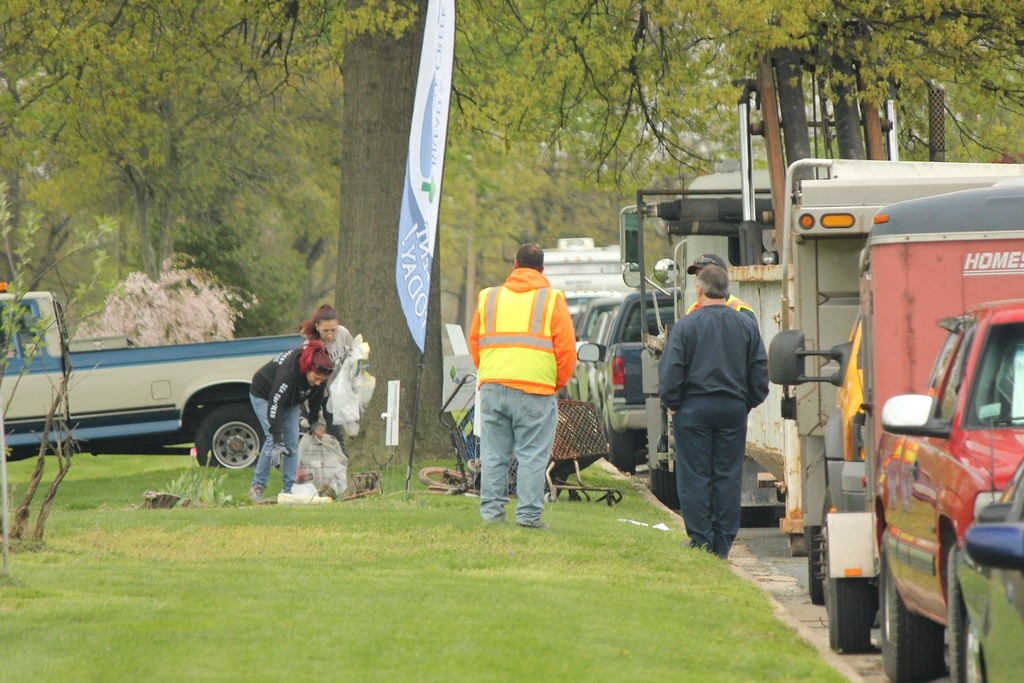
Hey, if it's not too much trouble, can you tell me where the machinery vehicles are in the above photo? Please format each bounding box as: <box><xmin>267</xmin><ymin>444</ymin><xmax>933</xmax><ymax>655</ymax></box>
<box><xmin>620</xmin><ymin>19</ymin><xmax>1024</xmax><ymax>683</ymax></box>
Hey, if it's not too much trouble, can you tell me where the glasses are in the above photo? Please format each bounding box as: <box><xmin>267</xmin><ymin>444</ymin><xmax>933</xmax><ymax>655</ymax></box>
<box><xmin>319</xmin><ymin>326</ymin><xmax>339</xmax><ymax>337</ymax></box>
<box><xmin>310</xmin><ymin>361</ymin><xmax>333</xmax><ymax>375</ymax></box>
<box><xmin>693</xmin><ymin>257</ymin><xmax>719</xmax><ymax>266</ymax></box>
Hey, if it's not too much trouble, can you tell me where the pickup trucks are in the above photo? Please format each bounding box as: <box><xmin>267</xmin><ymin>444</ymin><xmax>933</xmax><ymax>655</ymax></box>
<box><xmin>537</xmin><ymin>237</ymin><xmax>682</xmax><ymax>476</ymax></box>
<box><xmin>874</xmin><ymin>296</ymin><xmax>1022</xmax><ymax>682</ymax></box>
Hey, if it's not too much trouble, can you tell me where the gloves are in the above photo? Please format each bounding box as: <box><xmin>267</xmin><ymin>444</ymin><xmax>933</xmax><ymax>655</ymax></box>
<box><xmin>270</xmin><ymin>443</ymin><xmax>291</xmax><ymax>471</ymax></box>
<box><xmin>309</xmin><ymin>422</ymin><xmax>323</xmax><ymax>439</ymax></box>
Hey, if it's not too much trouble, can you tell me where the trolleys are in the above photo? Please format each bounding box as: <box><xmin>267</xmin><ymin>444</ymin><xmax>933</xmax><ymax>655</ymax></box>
<box><xmin>437</xmin><ymin>373</ymin><xmax>624</xmax><ymax>507</ymax></box>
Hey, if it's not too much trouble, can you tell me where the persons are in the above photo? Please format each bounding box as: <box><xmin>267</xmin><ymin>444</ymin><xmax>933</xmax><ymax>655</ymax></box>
<box><xmin>469</xmin><ymin>244</ymin><xmax>576</xmax><ymax>528</ymax></box>
<box><xmin>249</xmin><ymin>340</ymin><xmax>333</xmax><ymax>504</ymax></box>
<box><xmin>300</xmin><ymin>304</ymin><xmax>354</xmax><ymax>458</ymax></box>
<box><xmin>659</xmin><ymin>253</ymin><xmax>769</xmax><ymax>558</ymax></box>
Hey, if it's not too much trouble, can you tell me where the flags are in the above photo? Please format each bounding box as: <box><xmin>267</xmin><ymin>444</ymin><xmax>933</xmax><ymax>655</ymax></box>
<box><xmin>396</xmin><ymin>0</ymin><xmax>455</xmax><ymax>354</ymax></box>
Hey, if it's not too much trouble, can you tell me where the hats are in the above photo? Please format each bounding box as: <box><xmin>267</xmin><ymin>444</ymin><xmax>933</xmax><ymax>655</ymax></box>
<box><xmin>686</xmin><ymin>253</ymin><xmax>726</xmax><ymax>276</ymax></box>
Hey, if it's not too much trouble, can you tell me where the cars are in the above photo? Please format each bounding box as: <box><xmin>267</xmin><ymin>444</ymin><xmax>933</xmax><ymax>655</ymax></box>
<box><xmin>953</xmin><ymin>452</ymin><xmax>1024</xmax><ymax>683</ymax></box>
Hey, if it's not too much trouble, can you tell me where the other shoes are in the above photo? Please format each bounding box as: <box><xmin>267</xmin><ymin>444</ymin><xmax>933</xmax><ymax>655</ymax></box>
<box><xmin>250</xmin><ymin>484</ymin><xmax>262</xmax><ymax>504</ymax></box>
<box><xmin>519</xmin><ymin>524</ymin><xmax>548</xmax><ymax>529</ymax></box>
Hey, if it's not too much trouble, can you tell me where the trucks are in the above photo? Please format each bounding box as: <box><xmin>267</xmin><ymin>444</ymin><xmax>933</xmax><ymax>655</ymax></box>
<box><xmin>0</xmin><ymin>281</ymin><xmax>308</xmax><ymax>470</ymax></box>
